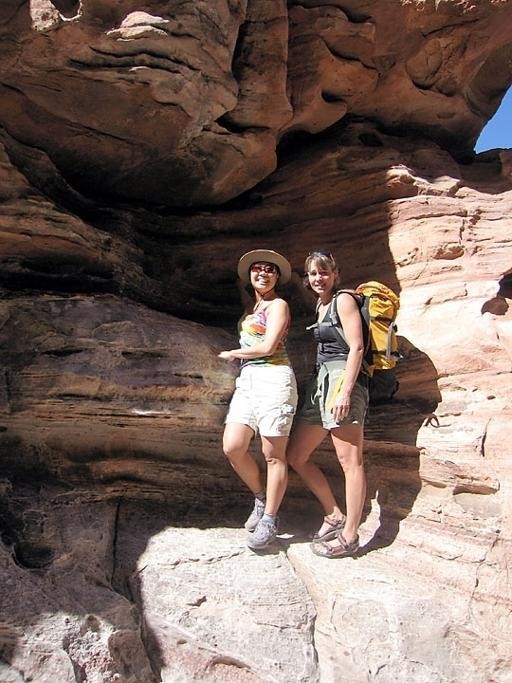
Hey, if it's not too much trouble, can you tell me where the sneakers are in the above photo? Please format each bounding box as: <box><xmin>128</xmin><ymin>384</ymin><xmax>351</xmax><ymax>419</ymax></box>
<box><xmin>244</xmin><ymin>495</ymin><xmax>266</xmax><ymax>532</ymax></box>
<box><xmin>247</xmin><ymin>516</ymin><xmax>280</xmax><ymax>549</ymax></box>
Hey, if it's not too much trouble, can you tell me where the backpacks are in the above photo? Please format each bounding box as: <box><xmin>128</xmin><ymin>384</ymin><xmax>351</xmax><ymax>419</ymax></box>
<box><xmin>305</xmin><ymin>280</ymin><xmax>404</xmax><ymax>419</ymax></box>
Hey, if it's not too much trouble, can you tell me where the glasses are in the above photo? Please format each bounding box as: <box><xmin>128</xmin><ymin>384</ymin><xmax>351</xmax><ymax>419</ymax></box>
<box><xmin>308</xmin><ymin>247</ymin><xmax>333</xmax><ymax>262</ymax></box>
<box><xmin>250</xmin><ymin>264</ymin><xmax>278</xmax><ymax>273</ymax></box>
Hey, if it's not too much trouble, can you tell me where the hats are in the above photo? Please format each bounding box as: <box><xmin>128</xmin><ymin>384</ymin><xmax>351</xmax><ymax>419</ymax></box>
<box><xmin>237</xmin><ymin>249</ymin><xmax>291</xmax><ymax>285</ymax></box>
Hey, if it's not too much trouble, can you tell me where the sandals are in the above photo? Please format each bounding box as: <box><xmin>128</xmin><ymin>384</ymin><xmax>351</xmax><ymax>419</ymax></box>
<box><xmin>304</xmin><ymin>514</ymin><xmax>346</xmax><ymax>543</ymax></box>
<box><xmin>309</xmin><ymin>530</ymin><xmax>360</xmax><ymax>558</ymax></box>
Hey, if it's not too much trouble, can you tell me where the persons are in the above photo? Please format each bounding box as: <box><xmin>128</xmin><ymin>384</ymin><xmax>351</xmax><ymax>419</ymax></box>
<box><xmin>288</xmin><ymin>250</ymin><xmax>371</xmax><ymax>559</ymax></box>
<box><xmin>216</xmin><ymin>249</ymin><xmax>302</xmax><ymax>550</ymax></box>
